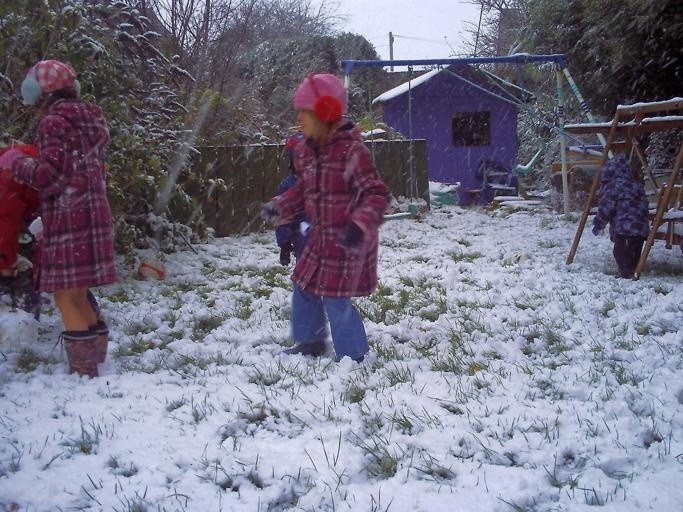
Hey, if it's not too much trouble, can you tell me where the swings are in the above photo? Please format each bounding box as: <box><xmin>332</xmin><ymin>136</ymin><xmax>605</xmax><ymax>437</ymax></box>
<box><xmin>365</xmin><ymin>61</ymin><xmax>427</xmax><ymax>213</ymax></box>
<box><xmin>436</xmin><ymin>59</ymin><xmax>610</xmax><ymax>161</ymax></box>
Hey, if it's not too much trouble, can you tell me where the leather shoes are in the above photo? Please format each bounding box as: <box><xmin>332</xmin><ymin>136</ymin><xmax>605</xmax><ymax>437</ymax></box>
<box><xmin>285</xmin><ymin>340</ymin><xmax>325</xmax><ymax>356</ymax></box>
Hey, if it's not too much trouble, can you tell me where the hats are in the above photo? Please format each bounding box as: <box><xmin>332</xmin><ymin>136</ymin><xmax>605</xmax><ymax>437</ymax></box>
<box><xmin>294</xmin><ymin>74</ymin><xmax>347</xmax><ymax>112</ymax></box>
<box><xmin>26</xmin><ymin>60</ymin><xmax>75</xmax><ymax>94</ymax></box>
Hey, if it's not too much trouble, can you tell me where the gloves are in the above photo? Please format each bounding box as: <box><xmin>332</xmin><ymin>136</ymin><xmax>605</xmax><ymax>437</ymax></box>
<box><xmin>279</xmin><ymin>243</ymin><xmax>293</xmax><ymax>266</ymax></box>
<box><xmin>0</xmin><ymin>150</ymin><xmax>23</xmax><ymax>170</ymax></box>
<box><xmin>335</xmin><ymin>223</ymin><xmax>362</xmax><ymax>251</ymax></box>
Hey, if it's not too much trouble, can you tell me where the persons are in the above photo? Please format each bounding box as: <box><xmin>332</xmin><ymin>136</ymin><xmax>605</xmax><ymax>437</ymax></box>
<box><xmin>258</xmin><ymin>72</ymin><xmax>393</xmax><ymax>364</ymax></box>
<box><xmin>0</xmin><ymin>59</ymin><xmax>120</xmax><ymax>376</ymax></box>
<box><xmin>591</xmin><ymin>153</ymin><xmax>652</xmax><ymax>279</ymax></box>
<box><xmin>272</xmin><ymin>132</ymin><xmax>326</xmax><ymax>270</ymax></box>
